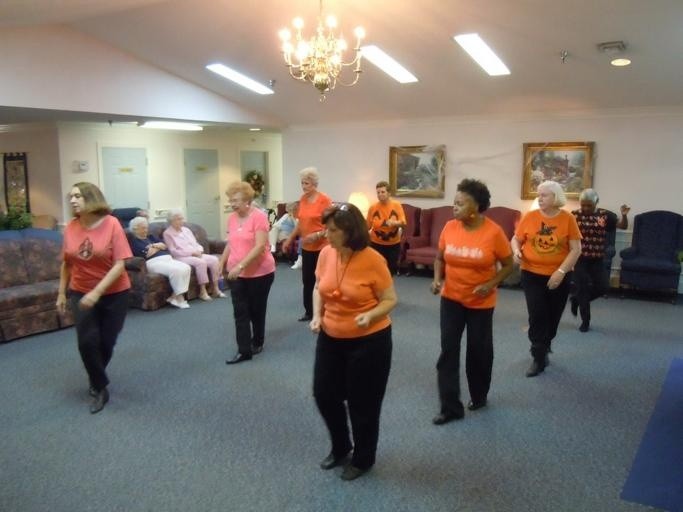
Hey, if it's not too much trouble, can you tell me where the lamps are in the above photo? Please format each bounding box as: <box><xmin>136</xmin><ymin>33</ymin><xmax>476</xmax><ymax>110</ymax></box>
<box><xmin>275</xmin><ymin>0</ymin><xmax>366</xmax><ymax>106</ymax></box>
<box><xmin>134</xmin><ymin>120</ymin><xmax>204</xmax><ymax>133</ymax></box>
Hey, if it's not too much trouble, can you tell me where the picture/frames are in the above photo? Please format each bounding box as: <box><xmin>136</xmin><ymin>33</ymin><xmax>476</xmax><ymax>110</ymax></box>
<box><xmin>520</xmin><ymin>142</ymin><xmax>595</xmax><ymax>201</ymax></box>
<box><xmin>387</xmin><ymin>144</ymin><xmax>445</xmax><ymax>200</ymax></box>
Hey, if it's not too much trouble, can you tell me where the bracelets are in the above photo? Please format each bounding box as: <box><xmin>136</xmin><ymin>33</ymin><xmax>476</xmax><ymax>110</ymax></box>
<box><xmin>557</xmin><ymin>266</ymin><xmax>568</xmax><ymax>275</ymax></box>
<box><xmin>238</xmin><ymin>264</ymin><xmax>243</xmax><ymax>270</ymax></box>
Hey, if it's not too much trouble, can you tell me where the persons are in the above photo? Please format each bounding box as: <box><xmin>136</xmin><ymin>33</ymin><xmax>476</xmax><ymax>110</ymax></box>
<box><xmin>429</xmin><ymin>177</ymin><xmax>515</xmax><ymax>425</ymax></box>
<box><xmin>282</xmin><ymin>170</ymin><xmax>332</xmax><ymax>322</ymax></box>
<box><xmin>53</xmin><ymin>182</ymin><xmax>135</xmax><ymax>415</ymax></box>
<box><xmin>511</xmin><ymin>181</ymin><xmax>582</xmax><ymax>377</ymax></box>
<box><xmin>217</xmin><ymin>179</ymin><xmax>277</xmax><ymax>364</ymax></box>
<box><xmin>128</xmin><ymin>216</ymin><xmax>192</xmax><ymax>309</ymax></box>
<box><xmin>568</xmin><ymin>188</ymin><xmax>632</xmax><ymax>333</ymax></box>
<box><xmin>268</xmin><ymin>201</ymin><xmax>303</xmax><ymax>269</ymax></box>
<box><xmin>366</xmin><ymin>181</ymin><xmax>406</xmax><ymax>277</ymax></box>
<box><xmin>308</xmin><ymin>202</ymin><xmax>400</xmax><ymax>480</ymax></box>
<box><xmin>163</xmin><ymin>208</ymin><xmax>227</xmax><ymax>300</ymax></box>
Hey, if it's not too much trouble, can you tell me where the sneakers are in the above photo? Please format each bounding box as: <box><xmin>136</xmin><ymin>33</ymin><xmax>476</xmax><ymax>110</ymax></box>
<box><xmin>171</xmin><ymin>300</ymin><xmax>190</xmax><ymax>308</ymax></box>
<box><xmin>291</xmin><ymin>261</ymin><xmax>302</xmax><ymax>270</ymax></box>
<box><xmin>166</xmin><ymin>297</ymin><xmax>187</xmax><ymax>304</ymax></box>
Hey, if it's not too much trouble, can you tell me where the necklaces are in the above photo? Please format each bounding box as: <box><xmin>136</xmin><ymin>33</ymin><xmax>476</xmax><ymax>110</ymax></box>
<box><xmin>333</xmin><ymin>249</ymin><xmax>354</xmax><ymax>297</ymax></box>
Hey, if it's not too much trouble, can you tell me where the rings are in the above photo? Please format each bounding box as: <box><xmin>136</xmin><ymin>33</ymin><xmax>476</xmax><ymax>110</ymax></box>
<box><xmin>554</xmin><ymin>281</ymin><xmax>558</xmax><ymax>285</ymax></box>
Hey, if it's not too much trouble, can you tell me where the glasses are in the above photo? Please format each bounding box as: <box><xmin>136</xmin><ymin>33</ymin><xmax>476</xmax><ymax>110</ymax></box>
<box><xmin>328</xmin><ymin>202</ymin><xmax>350</xmax><ymax>213</ymax></box>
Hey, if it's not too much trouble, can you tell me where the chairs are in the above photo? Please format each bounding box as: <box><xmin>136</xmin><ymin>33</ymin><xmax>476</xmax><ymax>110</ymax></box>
<box><xmin>619</xmin><ymin>211</ymin><xmax>682</xmax><ymax>306</ymax></box>
<box><xmin>405</xmin><ymin>206</ymin><xmax>454</xmax><ymax>275</ymax></box>
<box><xmin>396</xmin><ymin>202</ymin><xmax>420</xmax><ymax>274</ymax></box>
<box><xmin>601</xmin><ymin>208</ymin><xmax>617</xmax><ymax>299</ymax></box>
<box><xmin>482</xmin><ymin>207</ymin><xmax>521</xmax><ymax>242</ymax></box>
<box><xmin>275</xmin><ymin>203</ymin><xmax>294</xmax><ymax>266</ymax></box>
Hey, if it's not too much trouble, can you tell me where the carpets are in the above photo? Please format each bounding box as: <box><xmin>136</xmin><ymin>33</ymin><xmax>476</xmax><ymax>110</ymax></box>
<box><xmin>621</xmin><ymin>357</ymin><xmax>682</xmax><ymax>512</ymax></box>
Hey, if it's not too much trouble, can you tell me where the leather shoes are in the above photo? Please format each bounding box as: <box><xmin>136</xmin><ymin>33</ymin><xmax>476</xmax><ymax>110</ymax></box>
<box><xmin>569</xmin><ymin>296</ymin><xmax>578</xmax><ymax>316</ymax></box>
<box><xmin>526</xmin><ymin>362</ymin><xmax>544</xmax><ymax>377</ymax></box>
<box><xmin>468</xmin><ymin>400</ymin><xmax>480</xmax><ymax>410</ymax></box>
<box><xmin>320</xmin><ymin>454</ymin><xmax>337</xmax><ymax>469</ymax></box>
<box><xmin>90</xmin><ymin>385</ymin><xmax>98</xmax><ymax>396</ymax></box>
<box><xmin>340</xmin><ymin>461</ymin><xmax>372</xmax><ymax>480</ymax></box>
<box><xmin>251</xmin><ymin>345</ymin><xmax>263</xmax><ymax>354</ymax></box>
<box><xmin>579</xmin><ymin>320</ymin><xmax>590</xmax><ymax>332</ymax></box>
<box><xmin>432</xmin><ymin>411</ymin><xmax>452</xmax><ymax>425</ymax></box>
<box><xmin>91</xmin><ymin>391</ymin><xmax>110</xmax><ymax>413</ymax></box>
<box><xmin>547</xmin><ymin>342</ymin><xmax>551</xmax><ymax>353</ymax></box>
<box><xmin>297</xmin><ymin>313</ymin><xmax>311</xmax><ymax>321</ymax></box>
<box><xmin>226</xmin><ymin>352</ymin><xmax>252</xmax><ymax>364</ymax></box>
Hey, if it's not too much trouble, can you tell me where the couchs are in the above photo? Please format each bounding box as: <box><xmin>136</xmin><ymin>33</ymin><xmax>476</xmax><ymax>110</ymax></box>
<box><xmin>0</xmin><ymin>238</ymin><xmax>80</xmax><ymax>344</ymax></box>
<box><xmin>121</xmin><ymin>221</ymin><xmax>228</xmax><ymax>311</ymax></box>
